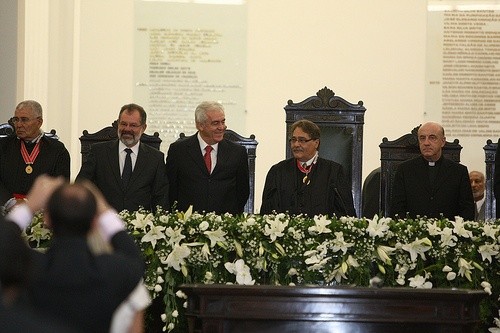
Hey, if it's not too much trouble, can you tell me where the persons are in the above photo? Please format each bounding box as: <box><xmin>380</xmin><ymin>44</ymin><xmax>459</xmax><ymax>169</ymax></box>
<box><xmin>166</xmin><ymin>100</ymin><xmax>251</xmax><ymax>217</ymax></box>
<box><xmin>469</xmin><ymin>171</ymin><xmax>487</xmax><ymax>221</ymax></box>
<box><xmin>260</xmin><ymin>119</ymin><xmax>357</xmax><ymax>218</ymax></box>
<box><xmin>391</xmin><ymin>122</ymin><xmax>478</xmax><ymax>221</ymax></box>
<box><xmin>0</xmin><ymin>100</ymin><xmax>170</xmax><ymax>333</ymax></box>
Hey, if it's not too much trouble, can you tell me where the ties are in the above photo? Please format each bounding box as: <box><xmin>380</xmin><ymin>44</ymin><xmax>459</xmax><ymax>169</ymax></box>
<box><xmin>122</xmin><ymin>149</ymin><xmax>133</xmax><ymax>185</ymax></box>
<box><xmin>302</xmin><ymin>163</ymin><xmax>308</xmax><ymax>170</ymax></box>
<box><xmin>475</xmin><ymin>201</ymin><xmax>479</xmax><ymax>220</ymax></box>
<box><xmin>27</xmin><ymin>141</ymin><xmax>33</xmax><ymax>154</ymax></box>
<box><xmin>204</xmin><ymin>146</ymin><xmax>214</xmax><ymax>174</ymax></box>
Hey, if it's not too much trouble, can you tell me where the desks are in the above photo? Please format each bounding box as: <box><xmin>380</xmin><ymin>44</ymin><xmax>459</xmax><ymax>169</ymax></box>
<box><xmin>146</xmin><ymin>282</ymin><xmax>500</xmax><ymax>332</ymax></box>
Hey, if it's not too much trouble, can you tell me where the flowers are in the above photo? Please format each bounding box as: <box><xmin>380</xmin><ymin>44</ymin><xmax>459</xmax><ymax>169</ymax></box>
<box><xmin>22</xmin><ymin>202</ymin><xmax>500</xmax><ymax>333</ymax></box>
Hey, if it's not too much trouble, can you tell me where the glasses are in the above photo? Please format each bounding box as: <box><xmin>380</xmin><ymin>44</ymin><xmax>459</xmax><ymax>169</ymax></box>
<box><xmin>118</xmin><ymin>122</ymin><xmax>143</xmax><ymax>128</ymax></box>
<box><xmin>289</xmin><ymin>138</ymin><xmax>313</xmax><ymax>143</ymax></box>
<box><xmin>13</xmin><ymin>117</ymin><xmax>40</xmax><ymax>122</ymax></box>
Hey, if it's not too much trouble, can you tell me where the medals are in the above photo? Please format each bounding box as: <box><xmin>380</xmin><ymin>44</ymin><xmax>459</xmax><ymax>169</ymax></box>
<box><xmin>303</xmin><ymin>176</ymin><xmax>311</xmax><ymax>185</ymax></box>
<box><xmin>25</xmin><ymin>165</ymin><xmax>33</xmax><ymax>174</ymax></box>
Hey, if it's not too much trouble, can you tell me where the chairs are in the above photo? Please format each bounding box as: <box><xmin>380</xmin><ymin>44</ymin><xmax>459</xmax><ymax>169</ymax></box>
<box><xmin>78</xmin><ymin>119</ymin><xmax>163</xmax><ymax>164</ymax></box>
<box><xmin>378</xmin><ymin>125</ymin><xmax>463</xmax><ymax>218</ymax></box>
<box><xmin>482</xmin><ymin>139</ymin><xmax>500</xmax><ymax>221</ymax></box>
<box><xmin>283</xmin><ymin>86</ymin><xmax>367</xmax><ymax>217</ymax></box>
<box><xmin>179</xmin><ymin>126</ymin><xmax>259</xmax><ymax>214</ymax></box>
